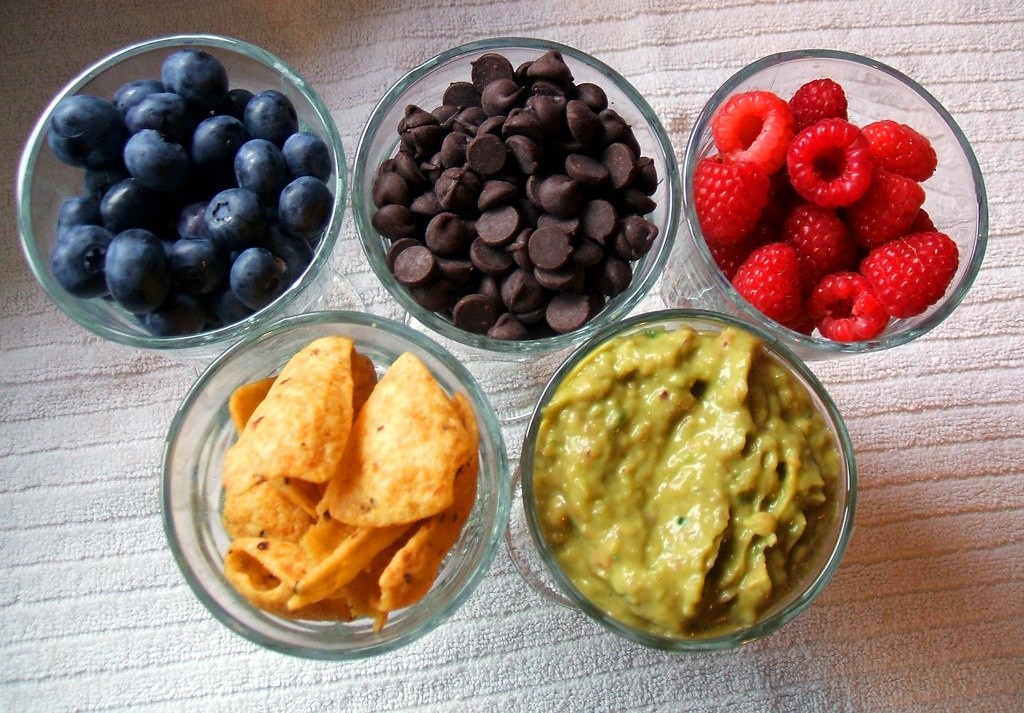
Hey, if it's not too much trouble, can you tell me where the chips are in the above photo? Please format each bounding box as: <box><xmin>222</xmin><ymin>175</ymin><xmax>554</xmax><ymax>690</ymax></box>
<box><xmin>228</xmin><ymin>335</ymin><xmax>479</xmax><ymax>623</ymax></box>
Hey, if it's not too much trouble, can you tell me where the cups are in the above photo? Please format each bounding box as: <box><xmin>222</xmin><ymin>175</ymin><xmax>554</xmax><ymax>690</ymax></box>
<box><xmin>157</xmin><ymin>309</ymin><xmax>510</xmax><ymax>662</ymax></box>
<box><xmin>13</xmin><ymin>32</ymin><xmax>350</xmax><ymax>362</ymax></box>
<box><xmin>510</xmin><ymin>306</ymin><xmax>860</xmax><ymax>654</ymax></box>
<box><xmin>661</xmin><ymin>47</ymin><xmax>990</xmax><ymax>363</ymax></box>
<box><xmin>349</xmin><ymin>35</ymin><xmax>685</xmax><ymax>357</ymax></box>
